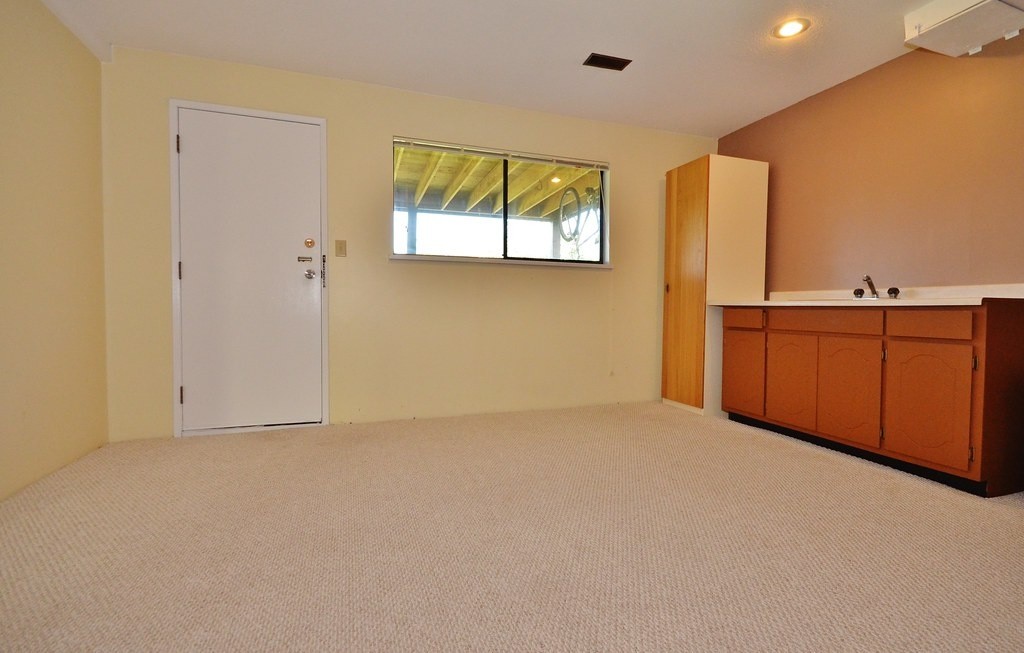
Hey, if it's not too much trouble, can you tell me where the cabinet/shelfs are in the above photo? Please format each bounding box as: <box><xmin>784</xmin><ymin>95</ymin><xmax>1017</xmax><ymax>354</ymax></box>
<box><xmin>708</xmin><ymin>297</ymin><xmax>1024</xmax><ymax>499</ymax></box>
<box><xmin>661</xmin><ymin>153</ymin><xmax>769</xmax><ymax>418</ymax></box>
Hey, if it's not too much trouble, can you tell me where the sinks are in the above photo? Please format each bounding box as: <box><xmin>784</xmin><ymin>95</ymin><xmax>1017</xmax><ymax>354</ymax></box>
<box><xmin>786</xmin><ymin>300</ymin><xmax>882</xmax><ymax>301</ymax></box>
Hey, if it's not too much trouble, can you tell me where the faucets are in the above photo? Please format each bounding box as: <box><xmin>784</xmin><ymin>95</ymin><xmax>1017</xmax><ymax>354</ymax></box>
<box><xmin>861</xmin><ymin>275</ymin><xmax>879</xmax><ymax>298</ymax></box>
